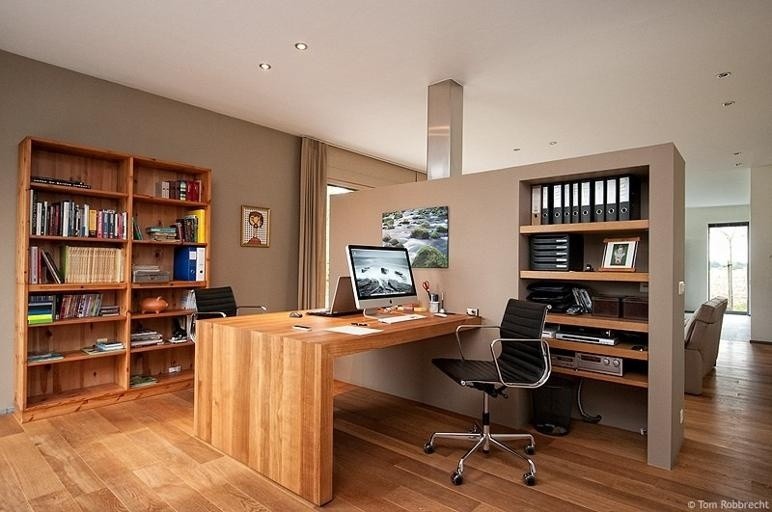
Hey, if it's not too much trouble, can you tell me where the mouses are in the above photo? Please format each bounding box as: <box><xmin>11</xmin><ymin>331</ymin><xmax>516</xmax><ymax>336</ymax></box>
<box><xmin>289</xmin><ymin>311</ymin><xmax>302</xmax><ymax>317</ymax></box>
<box><xmin>434</xmin><ymin>313</ymin><xmax>448</xmax><ymax>317</ymax></box>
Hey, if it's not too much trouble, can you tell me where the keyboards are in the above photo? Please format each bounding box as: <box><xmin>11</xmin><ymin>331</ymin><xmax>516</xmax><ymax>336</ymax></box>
<box><xmin>377</xmin><ymin>313</ymin><xmax>428</xmax><ymax>324</ymax></box>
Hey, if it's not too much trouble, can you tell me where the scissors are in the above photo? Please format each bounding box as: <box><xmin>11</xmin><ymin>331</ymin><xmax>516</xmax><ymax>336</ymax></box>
<box><xmin>422</xmin><ymin>281</ymin><xmax>431</xmax><ymax>301</ymax></box>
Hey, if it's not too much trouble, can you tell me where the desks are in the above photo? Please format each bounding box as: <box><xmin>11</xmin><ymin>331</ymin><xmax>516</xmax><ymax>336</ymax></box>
<box><xmin>193</xmin><ymin>304</ymin><xmax>480</xmax><ymax>507</ymax></box>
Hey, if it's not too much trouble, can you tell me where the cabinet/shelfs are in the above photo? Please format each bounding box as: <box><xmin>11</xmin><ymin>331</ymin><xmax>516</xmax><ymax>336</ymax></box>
<box><xmin>15</xmin><ymin>133</ymin><xmax>216</xmax><ymax>421</ymax></box>
<box><xmin>520</xmin><ymin>217</ymin><xmax>651</xmax><ymax>393</ymax></box>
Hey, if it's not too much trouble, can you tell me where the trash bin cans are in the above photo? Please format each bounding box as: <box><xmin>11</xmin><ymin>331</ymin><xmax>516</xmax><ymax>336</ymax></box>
<box><xmin>531</xmin><ymin>375</ymin><xmax>574</xmax><ymax>436</ymax></box>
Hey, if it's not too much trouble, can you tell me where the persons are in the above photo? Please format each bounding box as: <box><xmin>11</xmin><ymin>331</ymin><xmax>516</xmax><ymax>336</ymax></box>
<box><xmin>614</xmin><ymin>245</ymin><xmax>626</xmax><ymax>264</ymax></box>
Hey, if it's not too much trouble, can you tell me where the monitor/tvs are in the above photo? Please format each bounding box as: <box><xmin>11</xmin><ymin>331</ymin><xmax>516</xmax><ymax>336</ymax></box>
<box><xmin>345</xmin><ymin>244</ymin><xmax>419</xmax><ymax>320</ymax></box>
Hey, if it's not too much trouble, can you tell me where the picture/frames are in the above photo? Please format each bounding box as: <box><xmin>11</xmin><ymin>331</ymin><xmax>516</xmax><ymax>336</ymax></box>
<box><xmin>240</xmin><ymin>204</ymin><xmax>271</xmax><ymax>248</ymax></box>
<box><xmin>599</xmin><ymin>237</ymin><xmax>640</xmax><ymax>272</ymax></box>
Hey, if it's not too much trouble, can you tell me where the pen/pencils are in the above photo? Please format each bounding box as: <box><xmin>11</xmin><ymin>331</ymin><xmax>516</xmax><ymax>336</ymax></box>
<box><xmin>351</xmin><ymin>323</ymin><xmax>367</xmax><ymax>326</ymax></box>
<box><xmin>442</xmin><ymin>290</ymin><xmax>444</xmax><ymax>309</ymax></box>
<box><xmin>430</xmin><ymin>293</ymin><xmax>438</xmax><ymax>302</ymax></box>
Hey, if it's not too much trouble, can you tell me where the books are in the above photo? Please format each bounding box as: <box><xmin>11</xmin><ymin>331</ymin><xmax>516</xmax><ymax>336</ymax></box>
<box><xmin>130</xmin><ymin>374</ymin><xmax>159</xmax><ymax>388</ymax></box>
<box><xmin>27</xmin><ymin>177</ymin><xmax>206</xmax><ymax>367</ymax></box>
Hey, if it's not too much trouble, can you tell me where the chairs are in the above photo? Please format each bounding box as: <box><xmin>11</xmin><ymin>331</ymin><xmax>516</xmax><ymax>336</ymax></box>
<box><xmin>423</xmin><ymin>297</ymin><xmax>553</xmax><ymax>487</ymax></box>
<box><xmin>684</xmin><ymin>296</ymin><xmax>729</xmax><ymax>395</ymax></box>
<box><xmin>190</xmin><ymin>285</ymin><xmax>272</xmax><ymax>343</ymax></box>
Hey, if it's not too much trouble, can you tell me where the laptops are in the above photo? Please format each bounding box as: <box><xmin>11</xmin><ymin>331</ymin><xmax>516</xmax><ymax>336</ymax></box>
<box><xmin>307</xmin><ymin>276</ymin><xmax>363</xmax><ymax>317</ymax></box>
